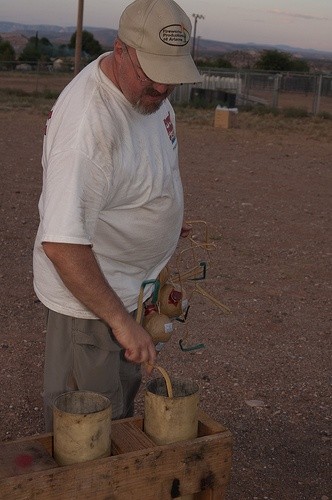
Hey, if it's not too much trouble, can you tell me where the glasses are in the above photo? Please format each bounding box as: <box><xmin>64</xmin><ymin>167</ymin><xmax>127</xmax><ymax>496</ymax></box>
<box><xmin>123</xmin><ymin>44</ymin><xmax>152</xmax><ymax>82</ymax></box>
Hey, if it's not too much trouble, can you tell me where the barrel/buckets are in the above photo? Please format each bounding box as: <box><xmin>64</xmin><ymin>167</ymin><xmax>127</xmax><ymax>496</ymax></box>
<box><xmin>51</xmin><ymin>391</ymin><xmax>112</xmax><ymax>465</ymax></box>
<box><xmin>144</xmin><ymin>377</ymin><xmax>200</xmax><ymax>446</ymax></box>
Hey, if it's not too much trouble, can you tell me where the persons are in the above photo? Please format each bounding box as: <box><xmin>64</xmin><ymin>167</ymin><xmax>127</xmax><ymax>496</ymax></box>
<box><xmin>32</xmin><ymin>0</ymin><xmax>204</xmax><ymax>432</ymax></box>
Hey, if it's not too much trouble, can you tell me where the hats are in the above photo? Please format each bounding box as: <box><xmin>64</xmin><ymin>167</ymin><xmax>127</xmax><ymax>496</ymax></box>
<box><xmin>117</xmin><ymin>0</ymin><xmax>204</xmax><ymax>85</ymax></box>
